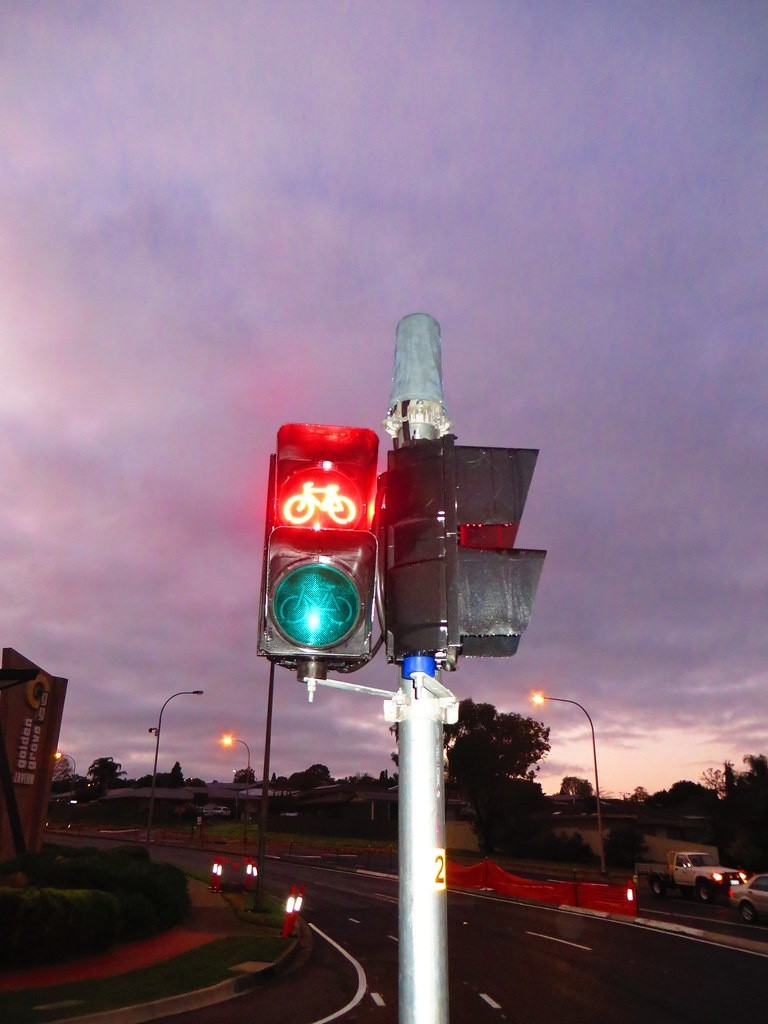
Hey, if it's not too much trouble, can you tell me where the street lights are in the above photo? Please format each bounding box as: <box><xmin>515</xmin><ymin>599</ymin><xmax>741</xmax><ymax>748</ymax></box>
<box><xmin>223</xmin><ymin>737</ymin><xmax>250</xmax><ymax>851</ymax></box>
<box><xmin>55</xmin><ymin>752</ymin><xmax>76</xmax><ymax>787</ymax></box>
<box><xmin>145</xmin><ymin>689</ymin><xmax>205</xmax><ymax>857</ymax></box>
<box><xmin>532</xmin><ymin>694</ymin><xmax>607</xmax><ymax>880</ymax></box>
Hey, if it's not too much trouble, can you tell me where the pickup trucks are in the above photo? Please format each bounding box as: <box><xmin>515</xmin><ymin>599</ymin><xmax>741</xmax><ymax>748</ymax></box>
<box><xmin>636</xmin><ymin>851</ymin><xmax>744</xmax><ymax>905</ymax></box>
<box><xmin>204</xmin><ymin>806</ymin><xmax>231</xmax><ymax>816</ymax></box>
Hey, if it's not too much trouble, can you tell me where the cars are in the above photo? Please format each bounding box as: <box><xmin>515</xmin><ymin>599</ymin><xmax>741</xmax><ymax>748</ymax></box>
<box><xmin>728</xmin><ymin>872</ymin><xmax>768</xmax><ymax>924</ymax></box>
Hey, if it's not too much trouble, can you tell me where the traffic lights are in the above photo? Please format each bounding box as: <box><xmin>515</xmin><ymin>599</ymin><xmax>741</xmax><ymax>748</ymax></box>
<box><xmin>452</xmin><ymin>443</ymin><xmax>547</xmax><ymax>660</ymax></box>
<box><xmin>267</xmin><ymin>423</ymin><xmax>385</xmax><ymax>667</ymax></box>
